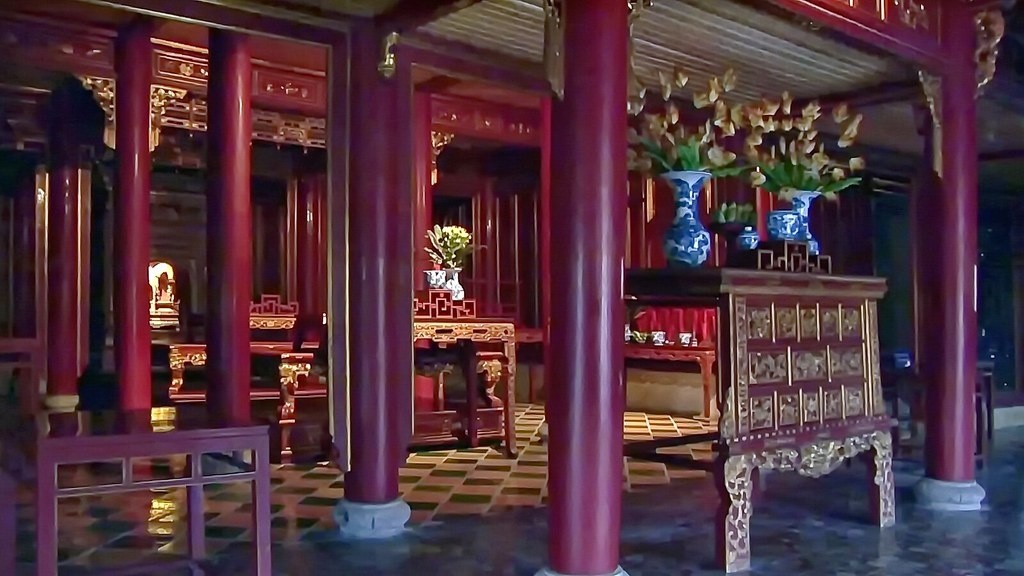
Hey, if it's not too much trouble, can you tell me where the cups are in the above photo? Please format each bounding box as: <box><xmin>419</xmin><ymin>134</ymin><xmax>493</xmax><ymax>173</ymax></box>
<box><xmin>422</xmin><ymin>270</ymin><xmax>447</xmax><ymax>288</ymax></box>
<box><xmin>652</xmin><ymin>330</ymin><xmax>666</xmax><ymax>346</ymax></box>
<box><xmin>679</xmin><ymin>333</ymin><xmax>692</xmax><ymax>346</ymax></box>
<box><xmin>767</xmin><ymin>210</ymin><xmax>801</xmax><ymax>242</ymax></box>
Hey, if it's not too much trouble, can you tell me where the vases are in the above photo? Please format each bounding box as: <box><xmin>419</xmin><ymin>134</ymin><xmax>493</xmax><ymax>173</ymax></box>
<box><xmin>792</xmin><ymin>190</ymin><xmax>822</xmax><ymax>256</ymax></box>
<box><xmin>658</xmin><ymin>171</ymin><xmax>713</xmax><ymax>266</ymax></box>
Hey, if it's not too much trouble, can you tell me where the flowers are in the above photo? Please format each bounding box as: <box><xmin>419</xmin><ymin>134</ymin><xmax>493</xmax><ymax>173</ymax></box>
<box><xmin>626</xmin><ymin>64</ymin><xmax>866</xmax><ymax>201</ymax></box>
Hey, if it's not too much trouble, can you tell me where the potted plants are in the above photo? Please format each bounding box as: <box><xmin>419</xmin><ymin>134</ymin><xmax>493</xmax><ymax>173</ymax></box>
<box><xmin>424</xmin><ymin>225</ymin><xmax>472</xmax><ymax>299</ymax></box>
<box><xmin>708</xmin><ymin>202</ymin><xmax>756</xmax><ymax>269</ymax></box>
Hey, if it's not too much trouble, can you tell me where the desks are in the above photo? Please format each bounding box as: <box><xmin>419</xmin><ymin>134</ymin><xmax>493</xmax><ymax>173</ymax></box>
<box><xmin>625</xmin><ymin>342</ymin><xmax>716</xmax><ymax>422</ymax></box>
<box><xmin>0</xmin><ymin>316</ymin><xmax>518</xmax><ymax>576</ymax></box>
<box><xmin>626</xmin><ymin>268</ymin><xmax>895</xmax><ymax>573</ymax></box>
<box><xmin>880</xmin><ymin>361</ymin><xmax>995</xmax><ymax>471</ymax></box>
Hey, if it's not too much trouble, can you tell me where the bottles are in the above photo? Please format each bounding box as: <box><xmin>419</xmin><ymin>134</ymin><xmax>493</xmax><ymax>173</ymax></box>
<box><xmin>736</xmin><ymin>226</ymin><xmax>759</xmax><ymax>252</ymax></box>
